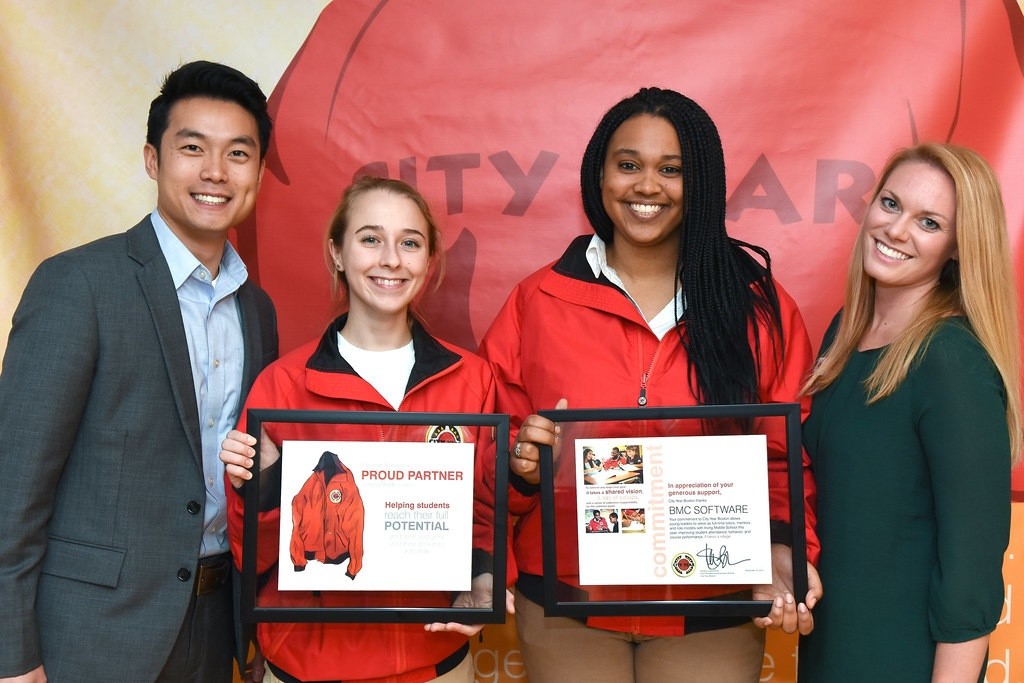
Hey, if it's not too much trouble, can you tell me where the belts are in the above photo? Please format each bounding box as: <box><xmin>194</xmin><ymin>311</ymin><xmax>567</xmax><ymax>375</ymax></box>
<box><xmin>193</xmin><ymin>558</ymin><xmax>233</xmax><ymax>596</ymax></box>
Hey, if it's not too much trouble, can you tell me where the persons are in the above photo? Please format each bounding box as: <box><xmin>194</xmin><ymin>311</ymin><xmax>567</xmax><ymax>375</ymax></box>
<box><xmin>584</xmin><ymin>446</ymin><xmax>645</xmax><ymax>533</ymax></box>
<box><xmin>797</xmin><ymin>143</ymin><xmax>1023</xmax><ymax>683</ymax></box>
<box><xmin>220</xmin><ymin>176</ymin><xmax>515</xmax><ymax>683</ymax></box>
<box><xmin>0</xmin><ymin>59</ymin><xmax>278</xmax><ymax>683</ymax></box>
<box><xmin>476</xmin><ymin>87</ymin><xmax>822</xmax><ymax>683</ymax></box>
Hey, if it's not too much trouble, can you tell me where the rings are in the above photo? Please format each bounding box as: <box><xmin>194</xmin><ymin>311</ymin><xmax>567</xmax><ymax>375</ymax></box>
<box><xmin>514</xmin><ymin>442</ymin><xmax>521</xmax><ymax>458</ymax></box>
